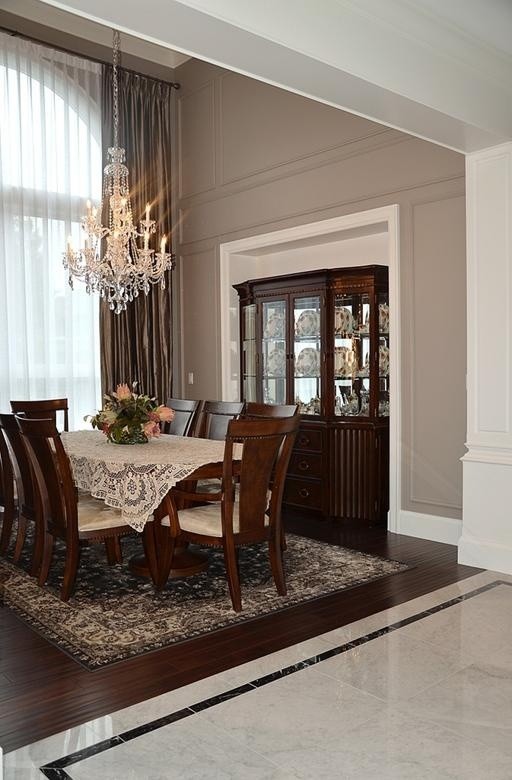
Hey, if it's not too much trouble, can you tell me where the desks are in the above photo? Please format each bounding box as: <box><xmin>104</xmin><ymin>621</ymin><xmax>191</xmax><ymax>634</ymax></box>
<box><xmin>49</xmin><ymin>431</ymin><xmax>244</xmax><ymax>577</ymax></box>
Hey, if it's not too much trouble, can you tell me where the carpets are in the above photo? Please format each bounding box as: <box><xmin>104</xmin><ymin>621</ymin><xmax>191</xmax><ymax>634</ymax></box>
<box><xmin>0</xmin><ymin>516</ymin><xmax>418</xmax><ymax>674</ymax></box>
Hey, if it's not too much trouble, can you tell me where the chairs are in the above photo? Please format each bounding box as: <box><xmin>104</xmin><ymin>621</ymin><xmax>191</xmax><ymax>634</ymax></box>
<box><xmin>156</xmin><ymin>415</ymin><xmax>301</xmax><ymax>610</ymax></box>
<box><xmin>238</xmin><ymin>402</ymin><xmax>299</xmax><ymax>550</ymax></box>
<box><xmin>197</xmin><ymin>401</ymin><xmax>245</xmax><ymax>440</ymax></box>
<box><xmin>159</xmin><ymin>399</ymin><xmax>202</xmax><ymax>437</ymax></box>
<box><xmin>0</xmin><ymin>397</ymin><xmax>69</xmax><ymax>551</ymax></box>
<box><xmin>1</xmin><ymin>413</ymin><xmax>121</xmax><ymax>576</ymax></box>
<box><xmin>13</xmin><ymin>415</ymin><xmax>160</xmax><ymax>603</ymax></box>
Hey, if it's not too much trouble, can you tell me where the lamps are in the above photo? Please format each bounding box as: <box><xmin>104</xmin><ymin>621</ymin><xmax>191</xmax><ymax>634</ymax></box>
<box><xmin>61</xmin><ymin>27</ymin><xmax>173</xmax><ymax>315</ymax></box>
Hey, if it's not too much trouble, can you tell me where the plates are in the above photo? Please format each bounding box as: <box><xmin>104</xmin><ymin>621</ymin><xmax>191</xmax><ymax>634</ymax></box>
<box><xmin>264</xmin><ymin>304</ymin><xmax>390</xmax><ymax>377</ymax></box>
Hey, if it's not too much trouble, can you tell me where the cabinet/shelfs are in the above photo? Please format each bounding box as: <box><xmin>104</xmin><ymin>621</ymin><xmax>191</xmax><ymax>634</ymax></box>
<box><xmin>231</xmin><ymin>263</ymin><xmax>390</xmax><ymax>526</ymax></box>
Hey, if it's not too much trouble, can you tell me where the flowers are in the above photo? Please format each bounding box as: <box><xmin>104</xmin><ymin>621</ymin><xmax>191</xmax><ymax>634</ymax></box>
<box><xmin>83</xmin><ymin>380</ymin><xmax>175</xmax><ymax>445</ymax></box>
